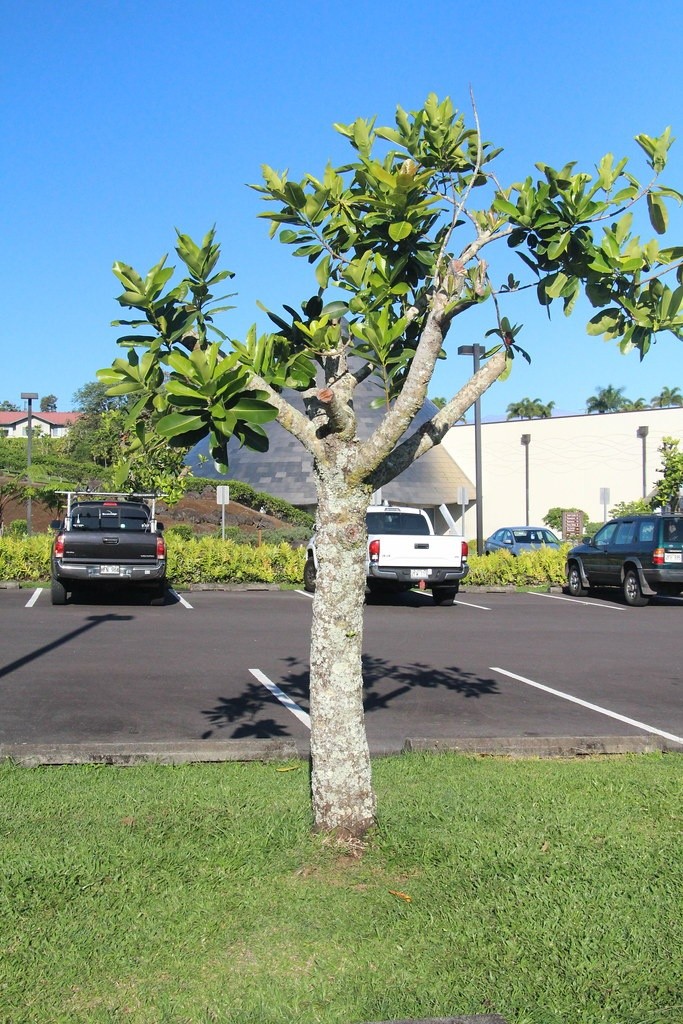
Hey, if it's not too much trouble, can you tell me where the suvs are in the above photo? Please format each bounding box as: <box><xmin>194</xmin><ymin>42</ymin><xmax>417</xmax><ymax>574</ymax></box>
<box><xmin>563</xmin><ymin>511</ymin><xmax>682</xmax><ymax>606</ymax></box>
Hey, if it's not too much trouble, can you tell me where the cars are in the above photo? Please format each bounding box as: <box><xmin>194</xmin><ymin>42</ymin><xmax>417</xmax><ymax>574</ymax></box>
<box><xmin>485</xmin><ymin>526</ymin><xmax>561</xmax><ymax>556</ymax></box>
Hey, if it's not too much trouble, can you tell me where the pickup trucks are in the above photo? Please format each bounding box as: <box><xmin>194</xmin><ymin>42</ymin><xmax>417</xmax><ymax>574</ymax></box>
<box><xmin>303</xmin><ymin>505</ymin><xmax>468</xmax><ymax>604</ymax></box>
<box><xmin>49</xmin><ymin>501</ymin><xmax>167</xmax><ymax>605</ymax></box>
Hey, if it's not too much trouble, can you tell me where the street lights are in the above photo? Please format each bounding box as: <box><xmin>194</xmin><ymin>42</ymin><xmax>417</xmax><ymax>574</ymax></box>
<box><xmin>457</xmin><ymin>343</ymin><xmax>486</xmax><ymax>557</ymax></box>
<box><xmin>21</xmin><ymin>391</ymin><xmax>38</xmax><ymax>536</ymax></box>
